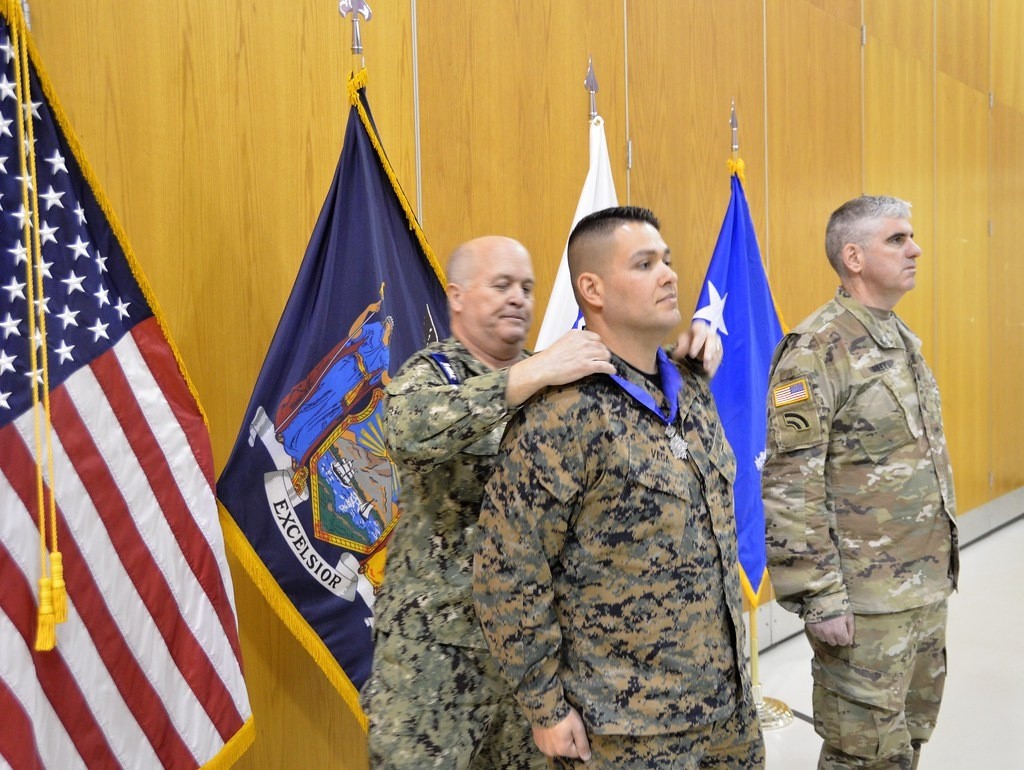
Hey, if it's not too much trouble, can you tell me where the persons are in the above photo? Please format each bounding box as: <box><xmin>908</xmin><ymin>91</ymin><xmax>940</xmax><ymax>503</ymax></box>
<box><xmin>362</xmin><ymin>232</ymin><xmax>624</xmax><ymax>769</ymax></box>
<box><xmin>473</xmin><ymin>207</ymin><xmax>768</xmax><ymax>770</ymax></box>
<box><xmin>764</xmin><ymin>195</ymin><xmax>966</xmax><ymax>770</ymax></box>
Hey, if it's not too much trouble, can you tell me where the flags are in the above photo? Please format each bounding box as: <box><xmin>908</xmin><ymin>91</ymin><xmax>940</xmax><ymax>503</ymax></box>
<box><xmin>525</xmin><ymin>111</ymin><xmax>624</xmax><ymax>398</ymax></box>
<box><xmin>0</xmin><ymin>8</ymin><xmax>274</xmax><ymax>770</ymax></box>
<box><xmin>680</xmin><ymin>159</ymin><xmax>802</xmax><ymax>612</ymax></box>
<box><xmin>214</xmin><ymin>65</ymin><xmax>458</xmax><ymax>735</ymax></box>
